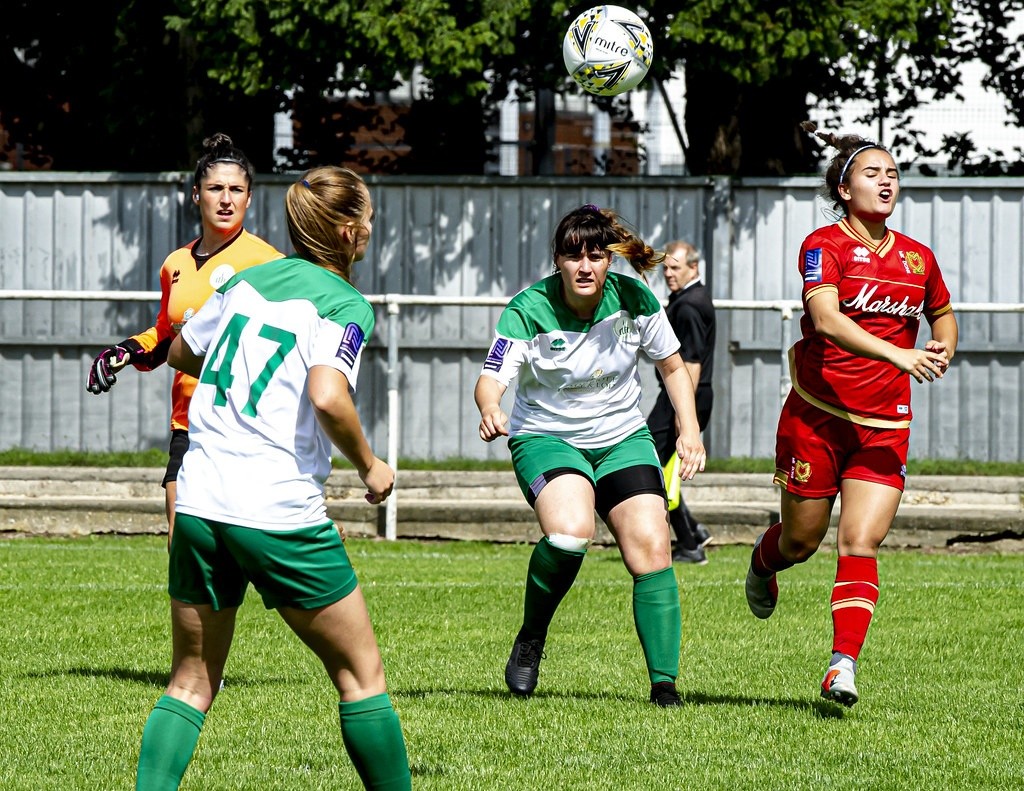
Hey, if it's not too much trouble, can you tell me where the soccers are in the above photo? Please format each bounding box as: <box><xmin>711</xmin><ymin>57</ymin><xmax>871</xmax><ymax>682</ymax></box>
<box><xmin>562</xmin><ymin>4</ymin><xmax>654</xmax><ymax>97</ymax></box>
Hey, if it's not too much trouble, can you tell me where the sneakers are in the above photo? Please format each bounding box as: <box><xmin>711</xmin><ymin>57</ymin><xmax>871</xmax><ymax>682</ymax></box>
<box><xmin>649</xmin><ymin>684</ymin><xmax>685</xmax><ymax>708</ymax></box>
<box><xmin>671</xmin><ymin>543</ymin><xmax>709</xmax><ymax>564</ymax></box>
<box><xmin>505</xmin><ymin>625</ymin><xmax>547</xmax><ymax>695</ymax></box>
<box><xmin>694</xmin><ymin>522</ymin><xmax>714</xmax><ymax>547</ymax></box>
<box><xmin>745</xmin><ymin>532</ymin><xmax>778</xmax><ymax>618</ymax></box>
<box><xmin>820</xmin><ymin>661</ymin><xmax>858</xmax><ymax>709</ymax></box>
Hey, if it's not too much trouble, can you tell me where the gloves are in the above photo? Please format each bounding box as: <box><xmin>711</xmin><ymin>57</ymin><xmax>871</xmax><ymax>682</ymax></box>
<box><xmin>86</xmin><ymin>338</ymin><xmax>144</xmax><ymax>395</ymax></box>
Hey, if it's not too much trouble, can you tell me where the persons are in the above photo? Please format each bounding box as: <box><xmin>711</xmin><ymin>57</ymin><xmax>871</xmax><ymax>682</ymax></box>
<box><xmin>129</xmin><ymin>167</ymin><xmax>412</xmax><ymax>791</ymax></box>
<box><xmin>746</xmin><ymin>142</ymin><xmax>959</xmax><ymax>713</ymax></box>
<box><xmin>82</xmin><ymin>135</ymin><xmax>291</xmax><ymax>554</ymax></box>
<box><xmin>473</xmin><ymin>202</ymin><xmax>707</xmax><ymax>710</ymax></box>
<box><xmin>644</xmin><ymin>239</ymin><xmax>720</xmax><ymax>566</ymax></box>
